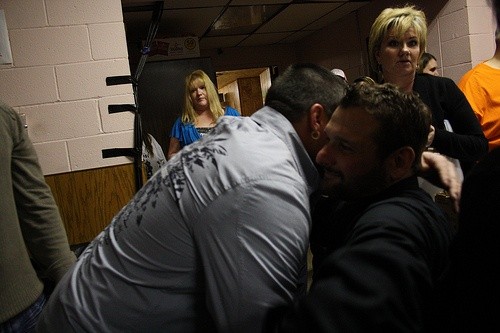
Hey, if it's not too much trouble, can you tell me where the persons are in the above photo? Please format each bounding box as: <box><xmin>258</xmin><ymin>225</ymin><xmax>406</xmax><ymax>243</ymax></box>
<box><xmin>256</xmin><ymin>76</ymin><xmax>500</xmax><ymax>333</ymax></box>
<box><xmin>170</xmin><ymin>70</ymin><xmax>238</xmax><ymax>163</ymax></box>
<box><xmin>456</xmin><ymin>1</ymin><xmax>500</xmax><ymax>159</ymax></box>
<box><xmin>35</xmin><ymin>61</ymin><xmax>465</xmax><ymax>333</ymax></box>
<box><xmin>0</xmin><ymin>102</ymin><xmax>76</xmax><ymax>333</ymax></box>
<box><xmin>140</xmin><ymin>127</ymin><xmax>168</xmax><ymax>186</ymax></box>
<box><xmin>415</xmin><ymin>51</ymin><xmax>439</xmax><ymax>77</ymax></box>
<box><xmin>363</xmin><ymin>5</ymin><xmax>498</xmax><ymax>222</ymax></box>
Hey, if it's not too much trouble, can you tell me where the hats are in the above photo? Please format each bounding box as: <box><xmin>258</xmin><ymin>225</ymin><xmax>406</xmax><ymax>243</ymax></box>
<box><xmin>330</xmin><ymin>68</ymin><xmax>349</xmax><ymax>83</ymax></box>
